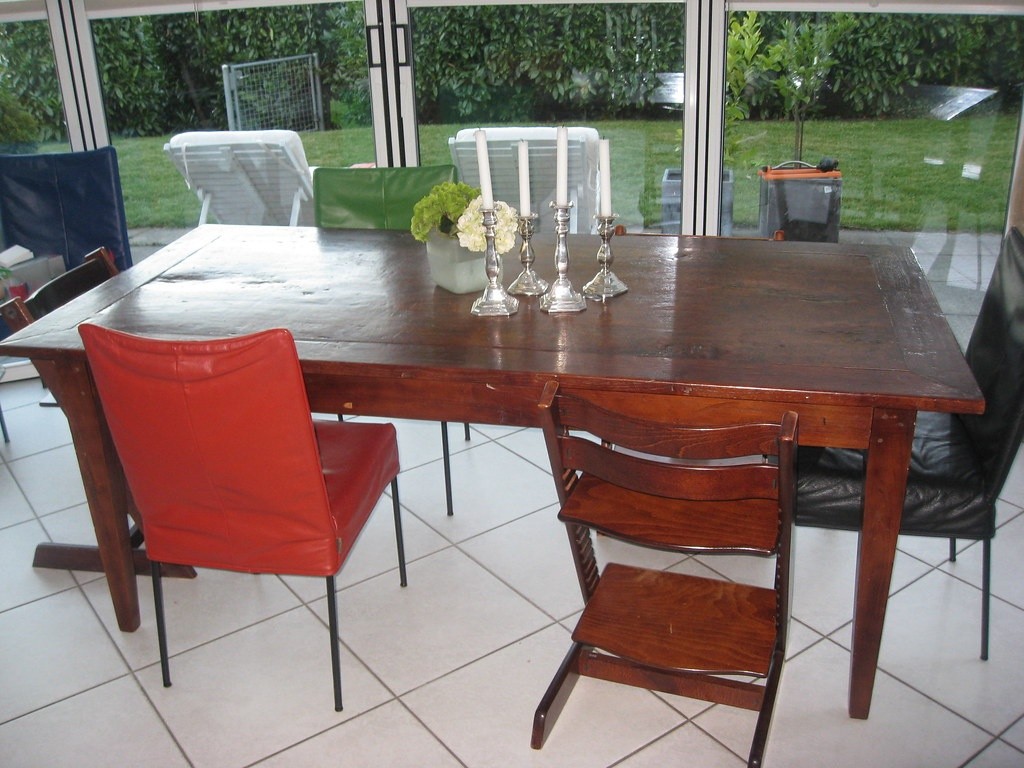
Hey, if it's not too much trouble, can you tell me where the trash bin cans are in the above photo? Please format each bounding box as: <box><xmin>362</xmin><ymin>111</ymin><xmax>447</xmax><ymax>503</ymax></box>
<box><xmin>660</xmin><ymin>168</ymin><xmax>734</xmax><ymax>237</ymax></box>
<box><xmin>757</xmin><ymin>158</ymin><xmax>843</xmax><ymax>243</ymax></box>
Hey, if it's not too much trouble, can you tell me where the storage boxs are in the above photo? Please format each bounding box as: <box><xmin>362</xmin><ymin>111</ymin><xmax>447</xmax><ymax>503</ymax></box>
<box><xmin>0</xmin><ymin>253</ymin><xmax>67</xmax><ymax>308</ymax></box>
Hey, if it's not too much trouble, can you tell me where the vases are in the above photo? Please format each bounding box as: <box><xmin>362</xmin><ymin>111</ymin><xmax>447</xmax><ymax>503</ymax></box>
<box><xmin>425</xmin><ymin>227</ymin><xmax>505</xmax><ymax>295</ymax></box>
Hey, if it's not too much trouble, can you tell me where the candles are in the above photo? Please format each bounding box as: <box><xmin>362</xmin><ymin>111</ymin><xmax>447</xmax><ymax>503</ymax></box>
<box><xmin>556</xmin><ymin>125</ymin><xmax>568</xmax><ymax>208</ymax></box>
<box><xmin>599</xmin><ymin>135</ymin><xmax>612</xmax><ymax>217</ymax></box>
<box><xmin>518</xmin><ymin>138</ymin><xmax>531</xmax><ymax>217</ymax></box>
<box><xmin>473</xmin><ymin>127</ymin><xmax>495</xmax><ymax>209</ymax></box>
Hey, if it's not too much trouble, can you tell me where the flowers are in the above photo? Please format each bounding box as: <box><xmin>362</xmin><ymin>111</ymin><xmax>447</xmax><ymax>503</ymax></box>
<box><xmin>409</xmin><ymin>180</ymin><xmax>517</xmax><ymax>254</ymax></box>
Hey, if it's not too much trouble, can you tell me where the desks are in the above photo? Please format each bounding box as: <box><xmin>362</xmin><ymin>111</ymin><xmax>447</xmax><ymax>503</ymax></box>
<box><xmin>0</xmin><ymin>225</ymin><xmax>986</xmax><ymax>719</ymax></box>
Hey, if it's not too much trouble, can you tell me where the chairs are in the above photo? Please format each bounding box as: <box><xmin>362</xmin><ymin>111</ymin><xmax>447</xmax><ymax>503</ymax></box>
<box><xmin>77</xmin><ymin>320</ymin><xmax>409</xmax><ymax>715</ymax></box>
<box><xmin>448</xmin><ymin>127</ymin><xmax>599</xmax><ymax>234</ymax></box>
<box><xmin>529</xmin><ymin>378</ymin><xmax>798</xmax><ymax>768</ymax></box>
<box><xmin>313</xmin><ymin>164</ymin><xmax>457</xmax><ymax>231</ymax></box>
<box><xmin>792</xmin><ymin>226</ymin><xmax>1024</xmax><ymax>663</ymax></box>
<box><xmin>164</xmin><ymin>130</ymin><xmax>377</xmax><ymax>227</ymax></box>
<box><xmin>0</xmin><ymin>247</ymin><xmax>199</xmax><ymax>578</ymax></box>
